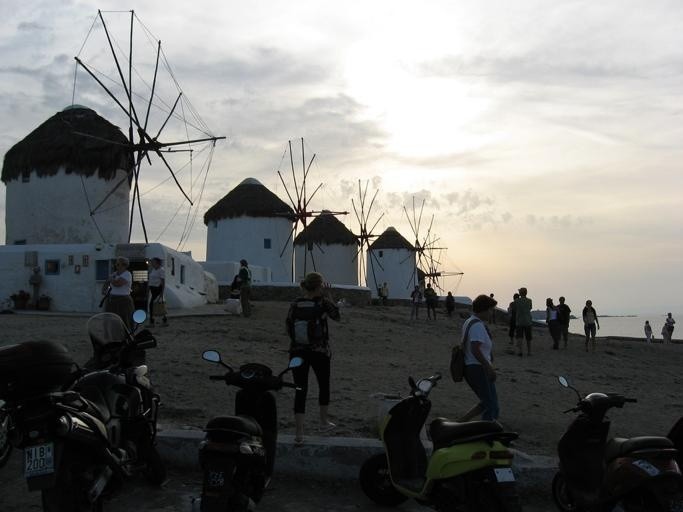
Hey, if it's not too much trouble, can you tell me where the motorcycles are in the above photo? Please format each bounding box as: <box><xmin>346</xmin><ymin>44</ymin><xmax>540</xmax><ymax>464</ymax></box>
<box><xmin>358</xmin><ymin>369</ymin><xmax>523</xmax><ymax>511</ymax></box>
<box><xmin>0</xmin><ymin>305</ymin><xmax>162</xmax><ymax>512</ymax></box>
<box><xmin>549</xmin><ymin>370</ymin><xmax>681</xmax><ymax>512</ymax></box>
<box><xmin>188</xmin><ymin>349</ymin><xmax>303</xmax><ymax>512</ymax></box>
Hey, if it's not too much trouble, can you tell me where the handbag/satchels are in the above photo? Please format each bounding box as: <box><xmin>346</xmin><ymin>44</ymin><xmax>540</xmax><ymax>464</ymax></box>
<box><xmin>151</xmin><ymin>293</ymin><xmax>167</xmax><ymax>316</ymax></box>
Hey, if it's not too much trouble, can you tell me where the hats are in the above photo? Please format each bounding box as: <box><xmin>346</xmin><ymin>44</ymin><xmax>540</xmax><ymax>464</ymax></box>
<box><xmin>471</xmin><ymin>293</ymin><xmax>498</xmax><ymax>313</ymax></box>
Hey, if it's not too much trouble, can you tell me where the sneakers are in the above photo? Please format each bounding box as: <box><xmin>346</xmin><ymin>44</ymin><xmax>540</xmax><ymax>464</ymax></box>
<box><xmin>292</xmin><ymin>438</ymin><xmax>304</xmax><ymax>447</ymax></box>
<box><xmin>317</xmin><ymin>421</ymin><xmax>336</xmax><ymax>433</ymax></box>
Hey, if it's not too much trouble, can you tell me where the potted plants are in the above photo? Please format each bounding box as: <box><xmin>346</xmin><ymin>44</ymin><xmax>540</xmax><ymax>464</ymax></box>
<box><xmin>10</xmin><ymin>290</ymin><xmax>30</xmax><ymax>309</ymax></box>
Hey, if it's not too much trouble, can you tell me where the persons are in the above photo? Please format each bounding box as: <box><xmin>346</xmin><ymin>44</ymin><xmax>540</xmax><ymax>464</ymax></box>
<box><xmin>102</xmin><ymin>257</ymin><xmax>135</xmax><ymax>341</ymax></box>
<box><xmin>231</xmin><ymin>274</ymin><xmax>255</xmax><ymax>308</ymax></box>
<box><xmin>661</xmin><ymin>323</ymin><xmax>668</xmax><ymax>344</ymax></box>
<box><xmin>458</xmin><ymin>294</ymin><xmax>499</xmax><ymax>422</ymax></box>
<box><xmin>240</xmin><ymin>259</ymin><xmax>252</xmax><ymax>317</ymax></box>
<box><xmin>285</xmin><ymin>271</ymin><xmax>340</xmax><ymax>445</ymax></box>
<box><xmin>30</xmin><ymin>267</ymin><xmax>41</xmax><ymax>303</ymax></box>
<box><xmin>644</xmin><ymin>320</ymin><xmax>652</xmax><ymax>344</ymax></box>
<box><xmin>666</xmin><ymin>313</ymin><xmax>675</xmax><ymax>342</ymax></box>
<box><xmin>583</xmin><ymin>300</ymin><xmax>600</xmax><ymax>351</ymax></box>
<box><xmin>378</xmin><ymin>282</ymin><xmax>571</xmax><ymax>356</ymax></box>
<box><xmin>146</xmin><ymin>258</ymin><xmax>169</xmax><ymax>328</ymax></box>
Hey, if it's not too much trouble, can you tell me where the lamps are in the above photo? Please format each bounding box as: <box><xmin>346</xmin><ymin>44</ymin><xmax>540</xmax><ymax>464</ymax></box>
<box><xmin>95</xmin><ymin>241</ymin><xmax>104</xmax><ymax>251</ymax></box>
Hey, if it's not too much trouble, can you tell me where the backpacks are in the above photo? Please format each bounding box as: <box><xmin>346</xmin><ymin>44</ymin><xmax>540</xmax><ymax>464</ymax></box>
<box><xmin>285</xmin><ymin>295</ymin><xmax>329</xmax><ymax>349</ymax></box>
<box><xmin>449</xmin><ymin>320</ymin><xmax>481</xmax><ymax>383</ymax></box>
